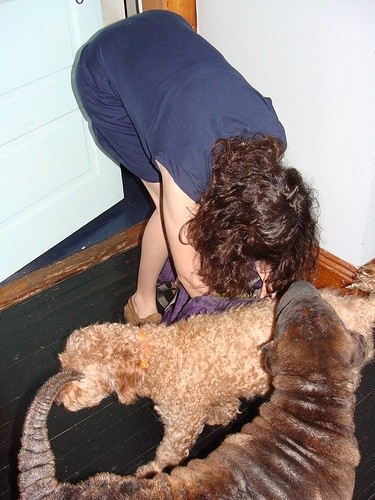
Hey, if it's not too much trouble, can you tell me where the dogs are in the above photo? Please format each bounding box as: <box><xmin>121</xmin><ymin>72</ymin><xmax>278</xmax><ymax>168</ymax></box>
<box><xmin>54</xmin><ymin>257</ymin><xmax>375</xmax><ymax>479</ymax></box>
<box><xmin>15</xmin><ymin>280</ymin><xmax>367</xmax><ymax>500</ymax></box>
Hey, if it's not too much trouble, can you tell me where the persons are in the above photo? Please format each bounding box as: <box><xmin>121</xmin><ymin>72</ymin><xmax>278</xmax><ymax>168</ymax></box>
<box><xmin>74</xmin><ymin>7</ymin><xmax>322</xmax><ymax>327</ymax></box>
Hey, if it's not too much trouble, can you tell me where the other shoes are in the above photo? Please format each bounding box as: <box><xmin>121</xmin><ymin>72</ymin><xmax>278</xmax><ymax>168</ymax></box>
<box><xmin>123</xmin><ymin>296</ymin><xmax>163</xmax><ymax>327</ymax></box>
